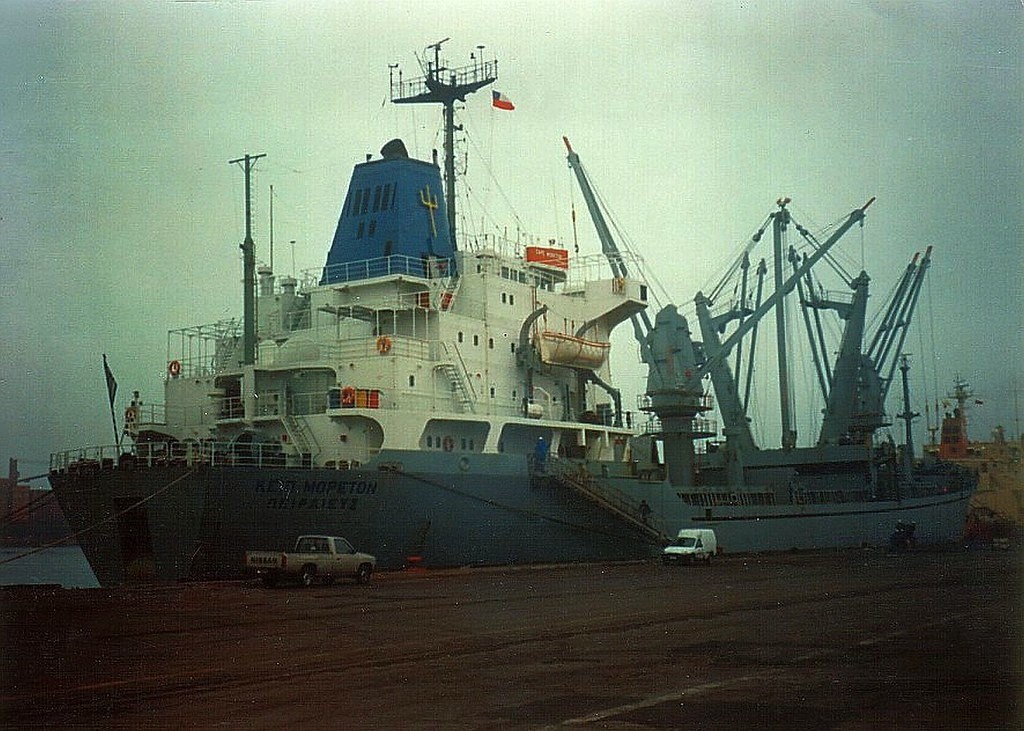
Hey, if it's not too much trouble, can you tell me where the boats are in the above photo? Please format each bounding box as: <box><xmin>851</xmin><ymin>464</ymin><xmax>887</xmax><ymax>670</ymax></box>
<box><xmin>530</xmin><ymin>327</ymin><xmax>613</xmax><ymax>371</ymax></box>
<box><xmin>921</xmin><ymin>369</ymin><xmax>1023</xmax><ymax>540</ymax></box>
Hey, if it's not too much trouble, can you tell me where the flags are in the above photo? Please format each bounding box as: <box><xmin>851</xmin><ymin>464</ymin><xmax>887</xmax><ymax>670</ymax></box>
<box><xmin>492</xmin><ymin>90</ymin><xmax>515</xmax><ymax>111</ymax></box>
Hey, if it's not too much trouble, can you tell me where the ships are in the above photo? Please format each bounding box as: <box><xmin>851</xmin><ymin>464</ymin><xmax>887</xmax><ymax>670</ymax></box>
<box><xmin>43</xmin><ymin>33</ymin><xmax>988</xmax><ymax>591</ymax></box>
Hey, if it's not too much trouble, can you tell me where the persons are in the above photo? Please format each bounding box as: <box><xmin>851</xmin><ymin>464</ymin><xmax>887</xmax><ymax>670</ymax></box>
<box><xmin>535</xmin><ymin>437</ymin><xmax>548</xmax><ymax>465</ymax></box>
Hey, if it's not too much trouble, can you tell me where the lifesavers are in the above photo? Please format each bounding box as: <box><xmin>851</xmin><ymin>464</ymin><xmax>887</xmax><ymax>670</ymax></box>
<box><xmin>375</xmin><ymin>335</ymin><xmax>392</xmax><ymax>354</ymax></box>
<box><xmin>168</xmin><ymin>360</ymin><xmax>181</xmax><ymax>375</ymax></box>
<box><xmin>340</xmin><ymin>385</ymin><xmax>355</xmax><ymax>406</ymax></box>
<box><xmin>124</xmin><ymin>406</ymin><xmax>137</xmax><ymax>422</ymax></box>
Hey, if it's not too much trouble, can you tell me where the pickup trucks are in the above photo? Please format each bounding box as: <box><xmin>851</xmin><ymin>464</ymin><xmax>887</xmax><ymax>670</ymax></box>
<box><xmin>245</xmin><ymin>533</ymin><xmax>379</xmax><ymax>588</ymax></box>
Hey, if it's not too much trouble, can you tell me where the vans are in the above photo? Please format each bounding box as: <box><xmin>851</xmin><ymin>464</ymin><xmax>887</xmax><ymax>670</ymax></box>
<box><xmin>664</xmin><ymin>525</ymin><xmax>718</xmax><ymax>567</ymax></box>
